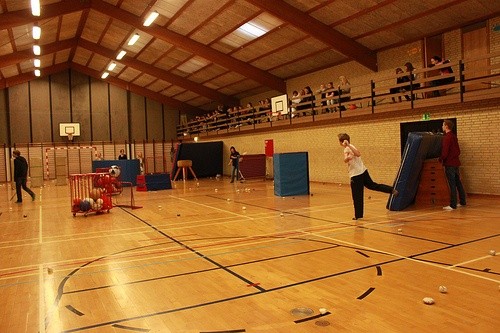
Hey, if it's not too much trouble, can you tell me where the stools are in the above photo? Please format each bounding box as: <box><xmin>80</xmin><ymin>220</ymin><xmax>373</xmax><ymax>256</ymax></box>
<box><xmin>173</xmin><ymin>160</ymin><xmax>198</xmax><ymax>183</ymax></box>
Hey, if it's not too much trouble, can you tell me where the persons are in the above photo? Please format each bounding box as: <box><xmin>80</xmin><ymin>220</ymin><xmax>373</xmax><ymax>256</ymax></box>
<box><xmin>136</xmin><ymin>149</ymin><xmax>147</xmax><ymax>175</ymax></box>
<box><xmin>96</xmin><ymin>152</ymin><xmax>103</xmax><ymax>160</ymax></box>
<box><xmin>438</xmin><ymin>117</ymin><xmax>468</xmax><ymax>211</ymax></box>
<box><xmin>174</xmin><ymin>75</ymin><xmax>352</xmax><ymax>138</ymax></box>
<box><xmin>390</xmin><ymin>62</ymin><xmax>421</xmax><ymax>103</ymax></box>
<box><xmin>227</xmin><ymin>147</ymin><xmax>240</xmax><ymax>183</ymax></box>
<box><xmin>428</xmin><ymin>55</ymin><xmax>455</xmax><ymax>97</ymax></box>
<box><xmin>118</xmin><ymin>148</ymin><xmax>128</xmax><ymax>160</ymax></box>
<box><xmin>12</xmin><ymin>149</ymin><xmax>36</xmax><ymax>204</ymax></box>
<box><xmin>338</xmin><ymin>131</ymin><xmax>401</xmax><ymax>220</ymax></box>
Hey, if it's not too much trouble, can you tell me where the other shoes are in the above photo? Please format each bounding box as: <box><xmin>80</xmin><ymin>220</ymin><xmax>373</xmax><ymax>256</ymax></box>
<box><xmin>394</xmin><ymin>192</ymin><xmax>399</xmax><ymax>199</ymax></box>
<box><xmin>352</xmin><ymin>218</ymin><xmax>358</xmax><ymax>220</ymax></box>
<box><xmin>443</xmin><ymin>205</ymin><xmax>453</xmax><ymax>209</ymax></box>
<box><xmin>15</xmin><ymin>201</ymin><xmax>22</xmax><ymax>203</ymax></box>
<box><xmin>457</xmin><ymin>203</ymin><xmax>462</xmax><ymax>207</ymax></box>
<box><xmin>32</xmin><ymin>194</ymin><xmax>35</xmax><ymax>201</ymax></box>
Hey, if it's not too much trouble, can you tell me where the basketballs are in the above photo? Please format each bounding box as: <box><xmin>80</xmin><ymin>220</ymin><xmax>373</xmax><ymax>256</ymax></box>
<box><xmin>72</xmin><ymin>174</ymin><xmax>110</xmax><ymax>211</ymax></box>
<box><xmin>110</xmin><ymin>177</ymin><xmax>123</xmax><ymax>192</ymax></box>
<box><xmin>108</xmin><ymin>165</ymin><xmax>121</xmax><ymax>177</ymax></box>
